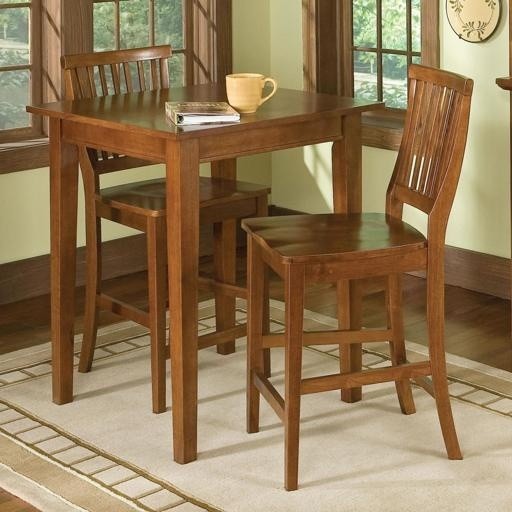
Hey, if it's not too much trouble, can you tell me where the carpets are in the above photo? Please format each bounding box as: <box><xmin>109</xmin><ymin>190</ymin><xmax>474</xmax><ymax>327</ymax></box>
<box><xmin>0</xmin><ymin>289</ymin><xmax>510</xmax><ymax>512</ymax></box>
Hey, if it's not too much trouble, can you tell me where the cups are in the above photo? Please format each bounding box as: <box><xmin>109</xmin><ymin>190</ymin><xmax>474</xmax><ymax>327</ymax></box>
<box><xmin>225</xmin><ymin>72</ymin><xmax>278</xmax><ymax>115</ymax></box>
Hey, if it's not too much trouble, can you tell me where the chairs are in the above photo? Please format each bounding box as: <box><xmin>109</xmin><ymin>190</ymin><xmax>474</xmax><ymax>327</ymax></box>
<box><xmin>62</xmin><ymin>43</ymin><xmax>268</xmax><ymax>411</ymax></box>
<box><xmin>238</xmin><ymin>61</ymin><xmax>477</xmax><ymax>490</ymax></box>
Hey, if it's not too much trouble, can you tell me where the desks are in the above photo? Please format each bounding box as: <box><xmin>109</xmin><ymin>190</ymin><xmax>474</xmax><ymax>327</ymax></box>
<box><xmin>25</xmin><ymin>78</ymin><xmax>390</xmax><ymax>465</ymax></box>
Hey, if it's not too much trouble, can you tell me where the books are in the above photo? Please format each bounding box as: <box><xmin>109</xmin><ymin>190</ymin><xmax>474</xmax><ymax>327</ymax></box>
<box><xmin>164</xmin><ymin>100</ymin><xmax>240</xmax><ymax>126</ymax></box>
